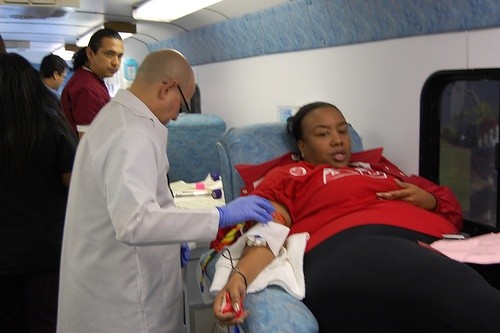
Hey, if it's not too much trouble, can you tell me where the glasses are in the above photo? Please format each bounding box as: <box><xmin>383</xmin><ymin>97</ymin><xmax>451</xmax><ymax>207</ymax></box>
<box><xmin>163</xmin><ymin>80</ymin><xmax>192</xmax><ymax>114</ymax></box>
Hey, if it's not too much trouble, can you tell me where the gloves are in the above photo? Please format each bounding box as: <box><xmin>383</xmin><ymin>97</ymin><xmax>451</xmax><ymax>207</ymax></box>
<box><xmin>181</xmin><ymin>242</ymin><xmax>190</xmax><ymax>268</ymax></box>
<box><xmin>216</xmin><ymin>194</ymin><xmax>274</xmax><ymax>227</ymax></box>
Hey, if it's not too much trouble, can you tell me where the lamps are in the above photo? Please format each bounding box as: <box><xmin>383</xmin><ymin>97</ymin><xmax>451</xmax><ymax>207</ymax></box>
<box><xmin>75</xmin><ymin>22</ymin><xmax>137</xmax><ymax>48</ymax></box>
<box><xmin>51</xmin><ymin>44</ymin><xmax>79</xmax><ymax>61</ymax></box>
<box><xmin>129</xmin><ymin>0</ymin><xmax>222</xmax><ymax>23</ymax></box>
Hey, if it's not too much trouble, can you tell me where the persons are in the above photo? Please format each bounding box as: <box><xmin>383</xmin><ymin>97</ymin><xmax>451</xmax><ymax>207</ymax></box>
<box><xmin>38</xmin><ymin>53</ymin><xmax>68</xmax><ymax>111</ymax></box>
<box><xmin>60</xmin><ymin>28</ymin><xmax>125</xmax><ymax>139</ymax></box>
<box><xmin>56</xmin><ymin>49</ymin><xmax>274</xmax><ymax>333</ymax></box>
<box><xmin>0</xmin><ymin>52</ymin><xmax>79</xmax><ymax>333</ymax></box>
<box><xmin>213</xmin><ymin>101</ymin><xmax>500</xmax><ymax>333</ymax></box>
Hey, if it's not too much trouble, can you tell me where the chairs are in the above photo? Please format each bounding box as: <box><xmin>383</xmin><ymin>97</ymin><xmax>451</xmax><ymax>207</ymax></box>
<box><xmin>164</xmin><ymin>112</ymin><xmax>364</xmax><ymax>333</ymax></box>
